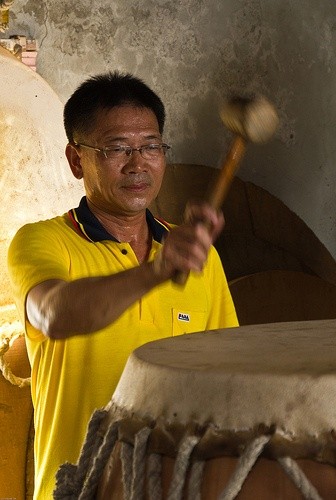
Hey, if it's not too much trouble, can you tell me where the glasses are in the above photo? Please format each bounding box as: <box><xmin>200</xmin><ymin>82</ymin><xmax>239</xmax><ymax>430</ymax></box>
<box><xmin>74</xmin><ymin>143</ymin><xmax>173</xmax><ymax>161</ymax></box>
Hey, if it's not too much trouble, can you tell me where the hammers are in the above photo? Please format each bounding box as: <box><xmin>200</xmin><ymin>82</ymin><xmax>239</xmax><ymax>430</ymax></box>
<box><xmin>171</xmin><ymin>87</ymin><xmax>278</xmax><ymax>288</ymax></box>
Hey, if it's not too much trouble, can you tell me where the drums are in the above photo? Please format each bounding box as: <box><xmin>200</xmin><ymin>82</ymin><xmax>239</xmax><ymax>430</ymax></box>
<box><xmin>73</xmin><ymin>316</ymin><xmax>336</xmax><ymax>499</ymax></box>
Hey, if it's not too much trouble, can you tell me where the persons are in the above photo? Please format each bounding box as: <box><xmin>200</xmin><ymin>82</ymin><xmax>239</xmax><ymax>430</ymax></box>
<box><xmin>7</xmin><ymin>71</ymin><xmax>234</xmax><ymax>500</ymax></box>
